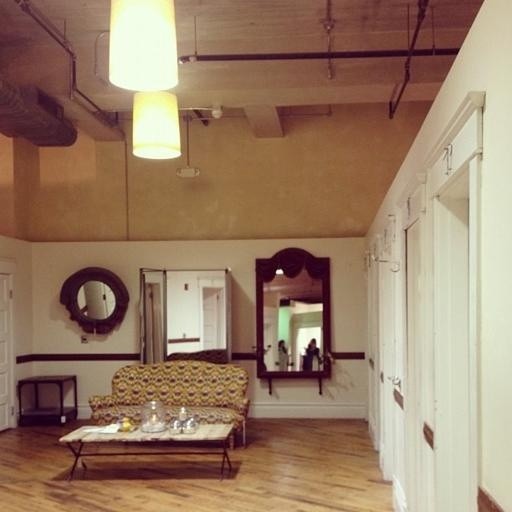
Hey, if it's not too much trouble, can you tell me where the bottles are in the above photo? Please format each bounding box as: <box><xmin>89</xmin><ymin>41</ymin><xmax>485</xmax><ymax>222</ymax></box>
<box><xmin>120</xmin><ymin>418</ymin><xmax>132</xmax><ymax>432</ymax></box>
<box><xmin>141</xmin><ymin>401</ymin><xmax>167</xmax><ymax>433</ymax></box>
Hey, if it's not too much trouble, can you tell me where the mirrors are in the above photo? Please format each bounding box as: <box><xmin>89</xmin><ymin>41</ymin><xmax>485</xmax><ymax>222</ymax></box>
<box><xmin>139</xmin><ymin>267</ymin><xmax>231</xmax><ymax>365</ymax></box>
<box><xmin>59</xmin><ymin>266</ymin><xmax>131</xmax><ymax>336</ymax></box>
<box><xmin>255</xmin><ymin>247</ymin><xmax>332</xmax><ymax>379</ymax></box>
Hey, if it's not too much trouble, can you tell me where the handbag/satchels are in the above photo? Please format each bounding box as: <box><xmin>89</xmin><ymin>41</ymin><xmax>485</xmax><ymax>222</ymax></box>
<box><xmin>318</xmin><ymin>356</ymin><xmax>323</xmax><ymax>366</ymax></box>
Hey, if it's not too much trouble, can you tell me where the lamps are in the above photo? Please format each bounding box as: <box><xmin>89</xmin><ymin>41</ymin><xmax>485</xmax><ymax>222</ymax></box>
<box><xmin>108</xmin><ymin>0</ymin><xmax>182</xmax><ymax>162</ymax></box>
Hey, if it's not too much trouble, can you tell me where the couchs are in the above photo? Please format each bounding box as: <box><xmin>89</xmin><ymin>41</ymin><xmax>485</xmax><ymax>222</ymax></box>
<box><xmin>87</xmin><ymin>360</ymin><xmax>253</xmax><ymax>450</ymax></box>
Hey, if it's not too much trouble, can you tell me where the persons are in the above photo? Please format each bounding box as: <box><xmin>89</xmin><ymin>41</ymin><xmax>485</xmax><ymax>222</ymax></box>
<box><xmin>278</xmin><ymin>339</ymin><xmax>294</xmax><ymax>371</ymax></box>
<box><xmin>301</xmin><ymin>337</ymin><xmax>321</xmax><ymax>371</ymax></box>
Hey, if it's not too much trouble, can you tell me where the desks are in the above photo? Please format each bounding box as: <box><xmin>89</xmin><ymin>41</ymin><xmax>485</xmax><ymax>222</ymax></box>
<box><xmin>16</xmin><ymin>375</ymin><xmax>78</xmax><ymax>427</ymax></box>
